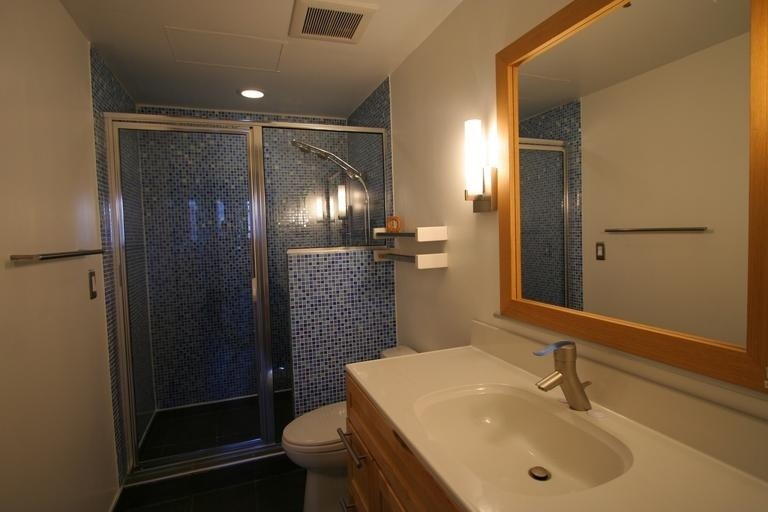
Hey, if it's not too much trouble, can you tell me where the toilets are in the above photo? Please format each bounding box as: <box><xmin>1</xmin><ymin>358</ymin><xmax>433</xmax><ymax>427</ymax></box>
<box><xmin>281</xmin><ymin>345</ymin><xmax>418</xmax><ymax>512</ymax></box>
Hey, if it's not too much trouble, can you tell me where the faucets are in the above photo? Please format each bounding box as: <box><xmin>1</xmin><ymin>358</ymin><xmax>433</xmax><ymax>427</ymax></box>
<box><xmin>532</xmin><ymin>341</ymin><xmax>591</xmax><ymax>410</ymax></box>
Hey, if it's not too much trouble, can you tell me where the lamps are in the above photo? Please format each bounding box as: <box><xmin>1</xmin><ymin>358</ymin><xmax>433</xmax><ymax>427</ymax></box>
<box><xmin>336</xmin><ymin>172</ymin><xmax>348</xmax><ymax>235</ymax></box>
<box><xmin>460</xmin><ymin>114</ymin><xmax>498</xmax><ymax>216</ymax></box>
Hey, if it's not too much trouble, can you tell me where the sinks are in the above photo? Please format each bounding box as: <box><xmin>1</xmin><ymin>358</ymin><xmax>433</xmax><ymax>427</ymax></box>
<box><xmin>413</xmin><ymin>384</ymin><xmax>633</xmax><ymax>499</ymax></box>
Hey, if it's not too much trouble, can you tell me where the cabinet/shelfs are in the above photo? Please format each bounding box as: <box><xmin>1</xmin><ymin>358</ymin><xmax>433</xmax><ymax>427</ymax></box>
<box><xmin>372</xmin><ymin>225</ymin><xmax>448</xmax><ymax>270</ymax></box>
<box><xmin>344</xmin><ymin>371</ymin><xmax>462</xmax><ymax>512</ymax></box>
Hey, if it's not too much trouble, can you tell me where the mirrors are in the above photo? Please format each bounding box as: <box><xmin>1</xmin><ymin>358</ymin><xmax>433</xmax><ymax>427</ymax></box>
<box><xmin>496</xmin><ymin>1</ymin><xmax>767</xmax><ymax>397</ymax></box>
